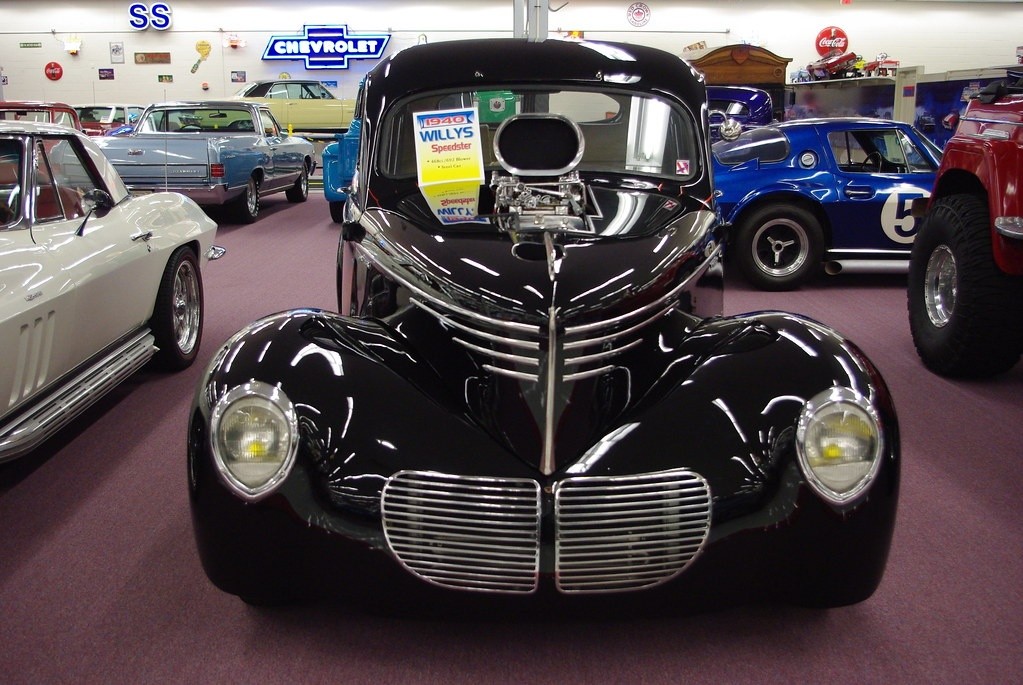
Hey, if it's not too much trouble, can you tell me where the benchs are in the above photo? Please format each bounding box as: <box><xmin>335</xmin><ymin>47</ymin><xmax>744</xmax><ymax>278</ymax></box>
<box><xmin>489</xmin><ymin>125</ymin><xmax>669</xmax><ymax>175</ymax></box>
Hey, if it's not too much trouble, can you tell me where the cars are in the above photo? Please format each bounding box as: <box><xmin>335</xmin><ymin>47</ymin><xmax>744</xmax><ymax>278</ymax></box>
<box><xmin>187</xmin><ymin>36</ymin><xmax>902</xmax><ymax>620</ymax></box>
<box><xmin>0</xmin><ymin>101</ymin><xmax>137</xmax><ymax>138</ymax></box>
<box><xmin>52</xmin><ymin>104</ymin><xmax>147</xmax><ymax>125</ymax></box>
<box><xmin>0</xmin><ymin>120</ymin><xmax>227</xmax><ymax>462</ymax></box>
<box><xmin>709</xmin><ymin>116</ymin><xmax>941</xmax><ymax>292</ymax></box>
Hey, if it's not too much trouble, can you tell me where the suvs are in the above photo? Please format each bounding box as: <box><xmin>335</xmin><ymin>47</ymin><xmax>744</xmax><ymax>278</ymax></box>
<box><xmin>908</xmin><ymin>69</ymin><xmax>1023</xmax><ymax>379</ymax></box>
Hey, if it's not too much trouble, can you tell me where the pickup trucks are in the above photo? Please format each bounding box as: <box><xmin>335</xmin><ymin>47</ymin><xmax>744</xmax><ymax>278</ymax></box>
<box><xmin>51</xmin><ymin>100</ymin><xmax>318</xmax><ymax>224</ymax></box>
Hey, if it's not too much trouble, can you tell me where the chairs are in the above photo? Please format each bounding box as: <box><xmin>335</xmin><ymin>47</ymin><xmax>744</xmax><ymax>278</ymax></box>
<box><xmin>37</xmin><ymin>186</ymin><xmax>81</xmax><ymax>219</ymax></box>
<box><xmin>227</xmin><ymin>119</ymin><xmax>254</xmax><ymax>130</ymax></box>
